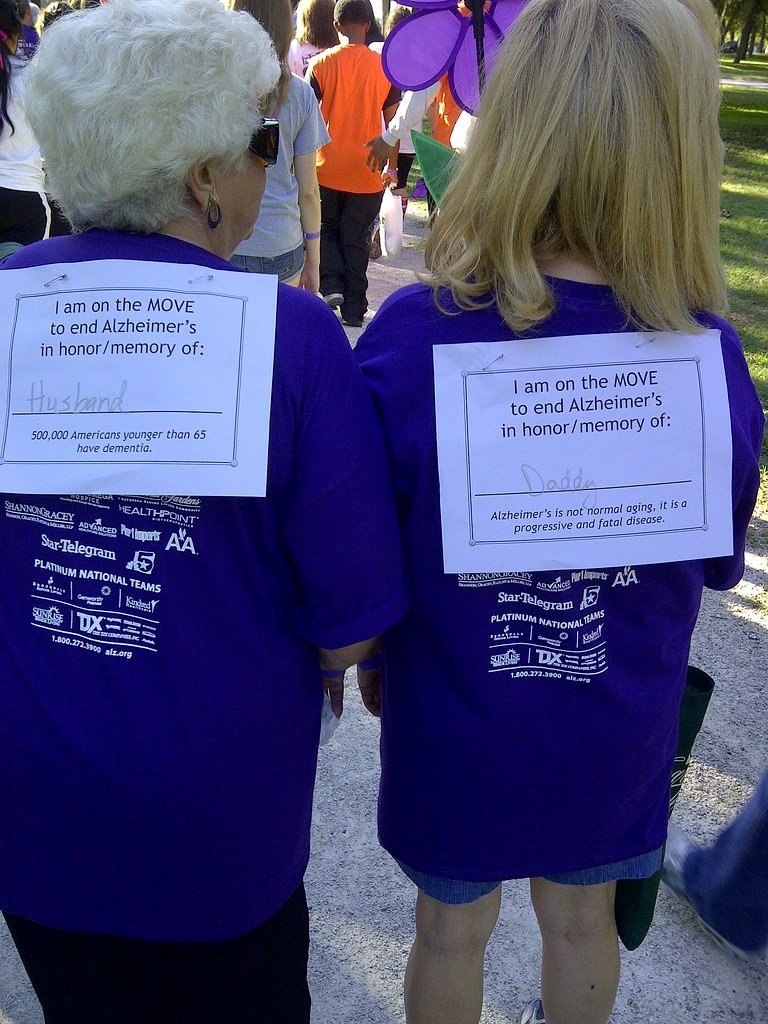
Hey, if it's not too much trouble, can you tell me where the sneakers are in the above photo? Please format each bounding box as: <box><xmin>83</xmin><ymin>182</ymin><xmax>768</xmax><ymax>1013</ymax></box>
<box><xmin>521</xmin><ymin>998</ymin><xmax>546</xmax><ymax>1022</ymax></box>
<box><xmin>655</xmin><ymin>826</ymin><xmax>765</xmax><ymax>966</ymax></box>
<box><xmin>344</xmin><ymin>315</ymin><xmax>363</xmax><ymax>328</ymax></box>
<box><xmin>323</xmin><ymin>292</ymin><xmax>344</xmax><ymax>312</ymax></box>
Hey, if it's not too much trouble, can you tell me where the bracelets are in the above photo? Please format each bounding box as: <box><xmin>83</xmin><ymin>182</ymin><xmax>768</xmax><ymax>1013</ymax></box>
<box><xmin>319</xmin><ymin>664</ymin><xmax>346</xmax><ymax>680</ymax></box>
<box><xmin>355</xmin><ymin>654</ymin><xmax>382</xmax><ymax>671</ymax></box>
<box><xmin>386</xmin><ymin>167</ymin><xmax>399</xmax><ymax>176</ymax></box>
<box><xmin>303</xmin><ymin>229</ymin><xmax>321</xmax><ymax>242</ymax></box>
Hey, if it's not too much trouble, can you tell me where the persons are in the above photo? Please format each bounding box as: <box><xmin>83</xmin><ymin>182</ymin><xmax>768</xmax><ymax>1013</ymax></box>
<box><xmin>0</xmin><ymin>11</ymin><xmax>56</xmax><ymax>249</ymax></box>
<box><xmin>229</xmin><ymin>1</ymin><xmax>331</xmax><ymax>298</ymax></box>
<box><xmin>17</xmin><ymin>2</ymin><xmax>384</xmax><ymax>73</ymax></box>
<box><xmin>305</xmin><ymin>2</ymin><xmax>399</xmax><ymax>326</ymax></box>
<box><xmin>362</xmin><ymin>0</ymin><xmax>492</xmax><ymax>230</ymax></box>
<box><xmin>650</xmin><ymin>773</ymin><xmax>767</xmax><ymax>958</ymax></box>
<box><xmin>0</xmin><ymin>1</ymin><xmax>414</xmax><ymax>1023</ymax></box>
<box><xmin>367</xmin><ymin>7</ymin><xmax>418</xmax><ymax>259</ymax></box>
<box><xmin>354</xmin><ymin>0</ymin><xmax>767</xmax><ymax>1024</ymax></box>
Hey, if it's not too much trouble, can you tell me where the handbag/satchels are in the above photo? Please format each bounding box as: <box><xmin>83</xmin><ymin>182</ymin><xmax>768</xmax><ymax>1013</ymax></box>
<box><xmin>616</xmin><ymin>665</ymin><xmax>712</xmax><ymax>952</ymax></box>
<box><xmin>381</xmin><ymin>186</ymin><xmax>403</xmax><ymax>259</ymax></box>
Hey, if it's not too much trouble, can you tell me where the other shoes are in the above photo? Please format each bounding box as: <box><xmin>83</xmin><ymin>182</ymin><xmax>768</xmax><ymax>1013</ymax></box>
<box><xmin>370</xmin><ymin>224</ymin><xmax>382</xmax><ymax>260</ymax></box>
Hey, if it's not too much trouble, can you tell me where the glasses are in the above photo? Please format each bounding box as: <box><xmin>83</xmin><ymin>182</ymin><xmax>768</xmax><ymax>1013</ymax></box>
<box><xmin>246</xmin><ymin>116</ymin><xmax>280</xmax><ymax>165</ymax></box>
